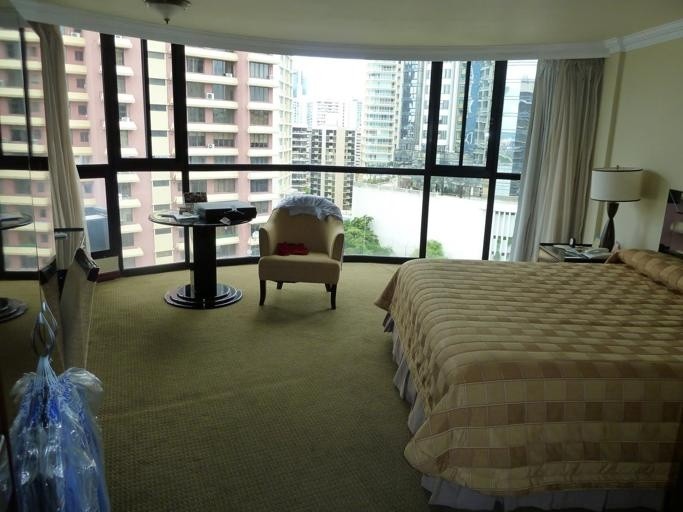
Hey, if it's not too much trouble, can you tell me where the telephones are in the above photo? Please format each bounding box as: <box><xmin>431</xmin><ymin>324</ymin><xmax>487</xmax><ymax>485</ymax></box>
<box><xmin>584</xmin><ymin>247</ymin><xmax>612</xmax><ymax>260</ymax></box>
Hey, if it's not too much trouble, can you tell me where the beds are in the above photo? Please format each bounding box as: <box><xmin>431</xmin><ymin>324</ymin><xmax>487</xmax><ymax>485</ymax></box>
<box><xmin>391</xmin><ymin>257</ymin><xmax>683</xmax><ymax>510</ymax></box>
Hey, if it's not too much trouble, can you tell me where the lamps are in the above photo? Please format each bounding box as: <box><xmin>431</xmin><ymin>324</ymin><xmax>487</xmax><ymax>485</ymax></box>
<box><xmin>590</xmin><ymin>164</ymin><xmax>643</xmax><ymax>252</ymax></box>
<box><xmin>144</xmin><ymin>0</ymin><xmax>191</xmax><ymax>25</ymax></box>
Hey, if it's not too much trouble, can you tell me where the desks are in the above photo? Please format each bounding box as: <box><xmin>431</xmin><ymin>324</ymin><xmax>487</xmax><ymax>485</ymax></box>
<box><xmin>0</xmin><ymin>212</ymin><xmax>33</xmax><ymax>322</ymax></box>
<box><xmin>148</xmin><ymin>208</ymin><xmax>251</xmax><ymax>309</ymax></box>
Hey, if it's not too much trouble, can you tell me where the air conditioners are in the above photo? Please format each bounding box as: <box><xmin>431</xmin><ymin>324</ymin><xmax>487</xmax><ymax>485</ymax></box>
<box><xmin>206</xmin><ymin>144</ymin><xmax>214</xmax><ymax>149</ymax></box>
<box><xmin>205</xmin><ymin>93</ymin><xmax>214</xmax><ymax>100</ymax></box>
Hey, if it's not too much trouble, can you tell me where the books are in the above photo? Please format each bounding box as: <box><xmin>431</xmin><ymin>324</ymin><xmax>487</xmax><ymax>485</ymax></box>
<box><xmin>158</xmin><ymin>210</ymin><xmax>179</xmax><ymax>217</ymax></box>
<box><xmin>0</xmin><ymin>210</ymin><xmax>22</xmax><ymax>222</ymax></box>
<box><xmin>172</xmin><ymin>212</ymin><xmax>199</xmax><ymax>221</ymax></box>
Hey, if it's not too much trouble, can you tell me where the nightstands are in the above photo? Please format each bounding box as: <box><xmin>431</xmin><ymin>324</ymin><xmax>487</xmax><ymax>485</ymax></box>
<box><xmin>536</xmin><ymin>242</ymin><xmax>606</xmax><ymax>263</ymax></box>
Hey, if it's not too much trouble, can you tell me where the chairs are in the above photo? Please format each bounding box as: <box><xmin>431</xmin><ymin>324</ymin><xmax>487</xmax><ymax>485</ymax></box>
<box><xmin>257</xmin><ymin>194</ymin><xmax>344</xmax><ymax>310</ymax></box>
